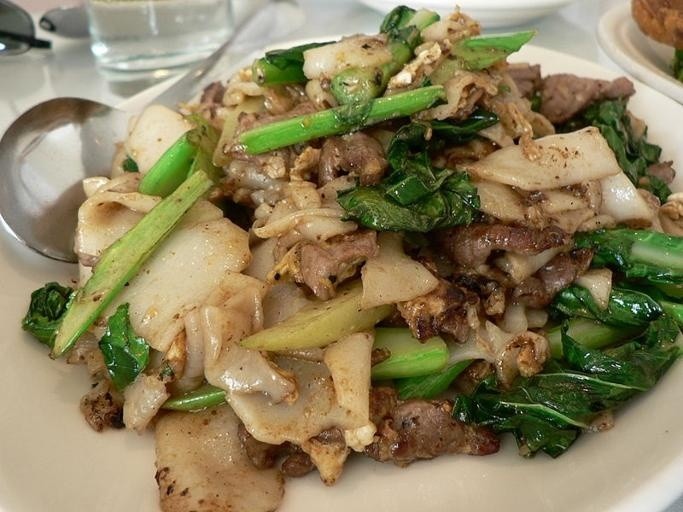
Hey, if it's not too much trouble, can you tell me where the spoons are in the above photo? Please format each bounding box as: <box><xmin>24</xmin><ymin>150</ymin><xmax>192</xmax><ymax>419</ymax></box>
<box><xmin>0</xmin><ymin>76</ymin><xmax>186</xmax><ymax>264</ymax></box>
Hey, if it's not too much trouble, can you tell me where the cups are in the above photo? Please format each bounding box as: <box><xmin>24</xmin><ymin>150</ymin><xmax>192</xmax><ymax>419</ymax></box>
<box><xmin>82</xmin><ymin>2</ymin><xmax>244</xmax><ymax>95</ymax></box>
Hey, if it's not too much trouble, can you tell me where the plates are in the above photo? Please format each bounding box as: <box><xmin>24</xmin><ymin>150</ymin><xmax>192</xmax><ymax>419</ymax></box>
<box><xmin>597</xmin><ymin>0</ymin><xmax>683</xmax><ymax>107</ymax></box>
<box><xmin>0</xmin><ymin>37</ymin><xmax>683</xmax><ymax>511</ymax></box>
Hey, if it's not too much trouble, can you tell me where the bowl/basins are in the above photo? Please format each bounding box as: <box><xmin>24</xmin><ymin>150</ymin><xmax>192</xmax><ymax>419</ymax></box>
<box><xmin>358</xmin><ymin>2</ymin><xmax>573</xmax><ymax>30</ymax></box>
